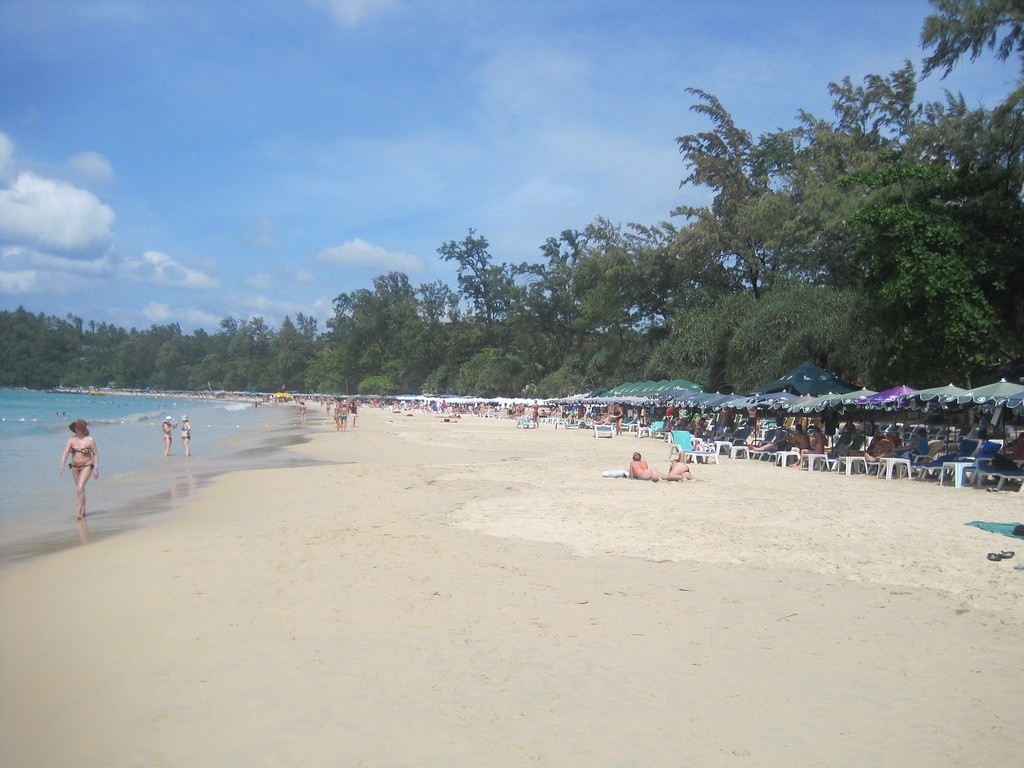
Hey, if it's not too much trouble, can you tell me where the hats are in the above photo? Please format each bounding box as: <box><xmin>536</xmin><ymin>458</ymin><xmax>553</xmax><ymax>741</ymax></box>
<box><xmin>179</xmin><ymin>415</ymin><xmax>189</xmax><ymax>421</ymax></box>
<box><xmin>69</xmin><ymin>420</ymin><xmax>90</xmax><ymax>436</ymax></box>
<box><xmin>164</xmin><ymin>416</ymin><xmax>175</xmax><ymax>424</ymax></box>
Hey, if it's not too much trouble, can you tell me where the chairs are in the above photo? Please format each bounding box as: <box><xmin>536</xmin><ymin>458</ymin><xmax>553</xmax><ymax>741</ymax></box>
<box><xmin>468</xmin><ymin>399</ymin><xmax>1024</xmax><ymax>497</ymax></box>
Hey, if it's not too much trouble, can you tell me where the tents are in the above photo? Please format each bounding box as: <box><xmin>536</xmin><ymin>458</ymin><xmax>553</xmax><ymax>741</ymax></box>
<box><xmin>720</xmin><ymin>361</ymin><xmax>1024</xmax><ymax>426</ymax></box>
<box><xmin>558</xmin><ymin>379</ymin><xmax>745</xmax><ymax>411</ymax></box>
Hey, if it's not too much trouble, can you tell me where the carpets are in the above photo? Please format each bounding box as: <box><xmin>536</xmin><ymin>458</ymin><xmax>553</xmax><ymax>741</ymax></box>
<box><xmin>964</xmin><ymin>520</ymin><xmax>1024</xmax><ymax>542</ymax></box>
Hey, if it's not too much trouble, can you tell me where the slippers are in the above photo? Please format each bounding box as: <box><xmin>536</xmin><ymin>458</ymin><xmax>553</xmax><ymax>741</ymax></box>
<box><xmin>987</xmin><ymin>553</ymin><xmax>1001</xmax><ymax>561</ymax></box>
<box><xmin>999</xmin><ymin>550</ymin><xmax>1015</xmax><ymax>559</ymax></box>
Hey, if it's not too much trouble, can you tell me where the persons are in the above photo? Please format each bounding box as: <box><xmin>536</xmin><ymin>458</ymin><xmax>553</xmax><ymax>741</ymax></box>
<box><xmin>163</xmin><ymin>416</ymin><xmax>177</xmax><ymax>456</ymax></box>
<box><xmin>661</xmin><ymin>451</ymin><xmax>694</xmax><ymax>482</ymax></box>
<box><xmin>326</xmin><ymin>396</ymin><xmax>357</xmax><ymax>432</ymax></box>
<box><xmin>975</xmin><ymin>412</ymin><xmax>994</xmax><ymax>439</ymax></box>
<box><xmin>595</xmin><ymin>402</ymin><xmax>623</xmax><ymax>437</ymax></box>
<box><xmin>510</xmin><ymin>402</ymin><xmax>525</xmax><ymax>417</ymax></box>
<box><xmin>60</xmin><ymin>419</ymin><xmax>99</xmax><ymax>519</ymax></box>
<box><xmin>393</xmin><ymin>399</ymin><xmax>447</xmax><ymax>414</ymax></box>
<box><xmin>753</xmin><ymin>424</ymin><xmax>824</xmax><ymax>469</ymax></box>
<box><xmin>531</xmin><ymin>400</ymin><xmax>539</xmax><ymax>428</ymax></box>
<box><xmin>630</xmin><ymin>452</ymin><xmax>685</xmax><ymax>483</ymax></box>
<box><xmin>660</xmin><ymin>417</ymin><xmax>708</xmax><ymax>439</ymax></box>
<box><xmin>300</xmin><ymin>402</ymin><xmax>306</xmax><ymax>417</ymax></box>
<box><xmin>842</xmin><ymin>418</ymin><xmax>928</xmax><ymax>462</ymax></box>
<box><xmin>180</xmin><ymin>415</ymin><xmax>190</xmax><ymax>457</ymax></box>
<box><xmin>477</xmin><ymin>402</ymin><xmax>492</xmax><ymax>416</ymax></box>
<box><xmin>1006</xmin><ymin>432</ymin><xmax>1024</xmax><ymax>465</ymax></box>
